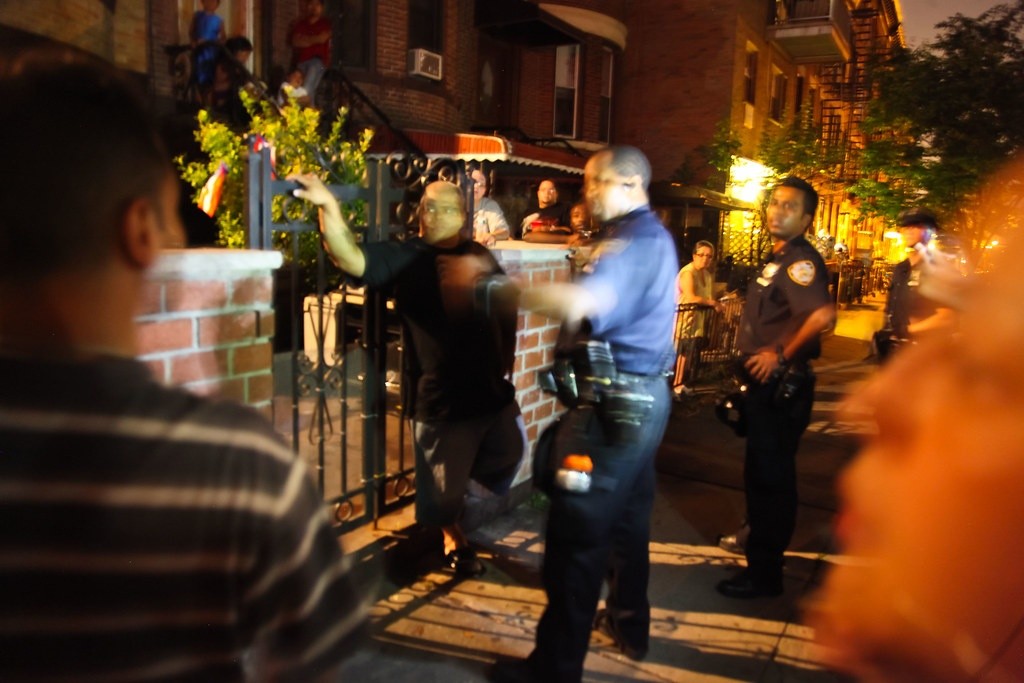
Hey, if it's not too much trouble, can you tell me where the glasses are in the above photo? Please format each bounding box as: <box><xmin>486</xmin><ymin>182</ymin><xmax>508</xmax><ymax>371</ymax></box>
<box><xmin>695</xmin><ymin>254</ymin><xmax>713</xmax><ymax>259</ymax></box>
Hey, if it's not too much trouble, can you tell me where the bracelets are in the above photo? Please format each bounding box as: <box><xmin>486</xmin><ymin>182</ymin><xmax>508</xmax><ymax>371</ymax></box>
<box><xmin>475</xmin><ymin>272</ymin><xmax>515</xmax><ymax>315</ymax></box>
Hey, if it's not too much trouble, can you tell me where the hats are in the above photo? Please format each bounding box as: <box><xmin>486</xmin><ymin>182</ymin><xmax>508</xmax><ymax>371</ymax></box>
<box><xmin>896</xmin><ymin>204</ymin><xmax>938</xmax><ymax>226</ymax></box>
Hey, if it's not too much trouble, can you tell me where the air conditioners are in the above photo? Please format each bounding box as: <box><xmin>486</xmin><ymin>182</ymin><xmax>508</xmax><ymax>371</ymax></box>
<box><xmin>407</xmin><ymin>48</ymin><xmax>442</xmax><ymax>81</ymax></box>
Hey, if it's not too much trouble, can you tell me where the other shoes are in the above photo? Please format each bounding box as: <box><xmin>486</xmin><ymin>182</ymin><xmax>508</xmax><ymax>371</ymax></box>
<box><xmin>590</xmin><ymin>610</ymin><xmax>649</xmax><ymax>663</ymax></box>
<box><xmin>489</xmin><ymin>658</ymin><xmax>532</xmax><ymax>683</ymax></box>
<box><xmin>443</xmin><ymin>547</ymin><xmax>488</xmax><ymax>579</ymax></box>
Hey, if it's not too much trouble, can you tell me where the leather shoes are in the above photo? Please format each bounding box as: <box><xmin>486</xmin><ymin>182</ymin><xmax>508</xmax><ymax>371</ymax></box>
<box><xmin>715</xmin><ymin>532</ymin><xmax>748</xmax><ymax>555</ymax></box>
<box><xmin>716</xmin><ymin>569</ymin><xmax>784</xmax><ymax>595</ymax></box>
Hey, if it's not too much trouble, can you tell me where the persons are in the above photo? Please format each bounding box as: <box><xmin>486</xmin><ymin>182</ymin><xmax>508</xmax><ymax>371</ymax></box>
<box><xmin>887</xmin><ymin>258</ymin><xmax>911</xmax><ymax>337</ymax></box>
<box><xmin>716</xmin><ymin>176</ymin><xmax>835</xmax><ymax>602</ymax></box>
<box><xmin>812</xmin><ymin>156</ymin><xmax>1024</xmax><ymax>683</ymax></box>
<box><xmin>439</xmin><ymin>145</ymin><xmax>680</xmax><ymax>682</ymax></box>
<box><xmin>0</xmin><ymin>63</ymin><xmax>377</xmax><ymax>683</ymax></box>
<box><xmin>188</xmin><ymin>0</ymin><xmax>598</xmax><ymax>248</ymax></box>
<box><xmin>675</xmin><ymin>241</ymin><xmax>725</xmax><ymax>396</ymax></box>
<box><xmin>285</xmin><ymin>172</ymin><xmax>517</xmax><ymax>574</ymax></box>
<box><xmin>835</xmin><ymin>239</ymin><xmax>848</xmax><ymax>258</ymax></box>
